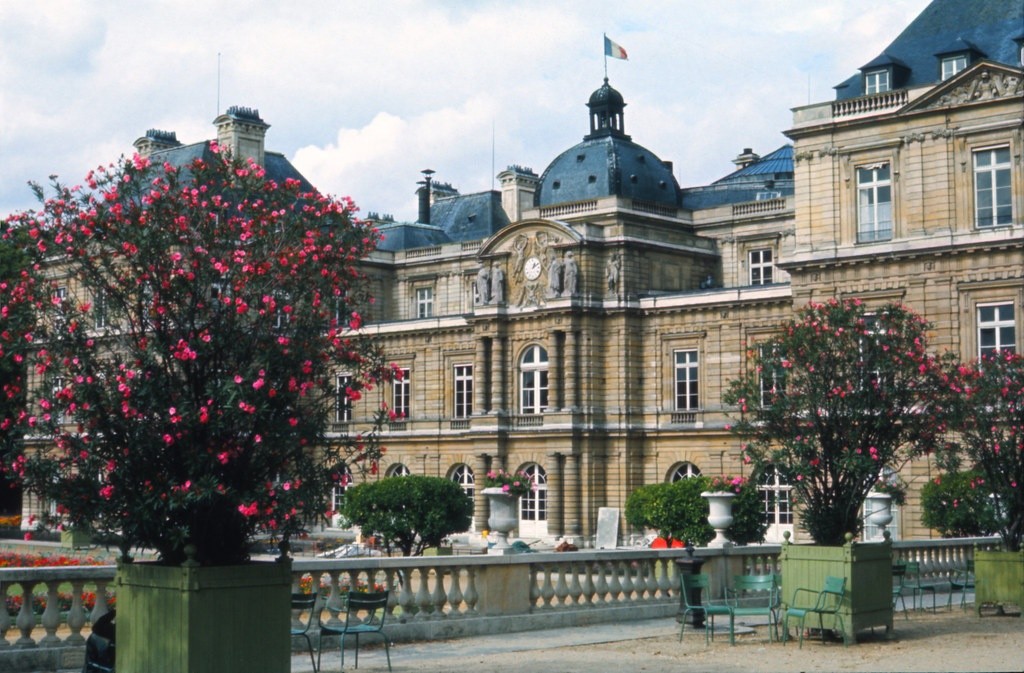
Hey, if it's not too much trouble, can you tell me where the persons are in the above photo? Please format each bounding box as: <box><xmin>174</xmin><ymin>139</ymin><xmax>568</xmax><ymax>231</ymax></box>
<box><xmin>544</xmin><ymin>255</ymin><xmax>560</xmax><ymax>291</ymax></box>
<box><xmin>491</xmin><ymin>262</ymin><xmax>504</xmax><ymax>302</ymax></box>
<box><xmin>535</xmin><ymin>234</ymin><xmax>548</xmax><ymax>272</ymax></box>
<box><xmin>520</xmin><ymin>283</ymin><xmax>540</xmax><ymax>310</ymax></box>
<box><xmin>560</xmin><ymin>251</ymin><xmax>578</xmax><ymax>296</ymax></box>
<box><xmin>477</xmin><ymin>264</ymin><xmax>489</xmax><ymax>303</ymax></box>
<box><xmin>606</xmin><ymin>253</ymin><xmax>621</xmax><ymax>291</ymax></box>
<box><xmin>512</xmin><ymin>238</ymin><xmax>527</xmax><ymax>277</ymax></box>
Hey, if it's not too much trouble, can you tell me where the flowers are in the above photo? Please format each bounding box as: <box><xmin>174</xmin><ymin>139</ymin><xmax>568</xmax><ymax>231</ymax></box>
<box><xmin>723</xmin><ymin>296</ymin><xmax>960</xmax><ymax>545</ymax></box>
<box><xmin>934</xmin><ymin>347</ymin><xmax>1024</xmax><ymax>551</ymax></box>
<box><xmin>486</xmin><ymin>469</ymin><xmax>537</xmax><ymax>496</ymax></box>
<box><xmin>0</xmin><ymin>139</ymin><xmax>406</xmax><ymax>547</ymax></box>
<box><xmin>704</xmin><ymin>476</ymin><xmax>750</xmax><ymax>493</ymax></box>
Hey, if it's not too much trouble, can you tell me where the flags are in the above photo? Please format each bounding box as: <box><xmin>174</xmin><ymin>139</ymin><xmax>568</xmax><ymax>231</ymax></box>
<box><xmin>604</xmin><ymin>37</ymin><xmax>627</xmax><ymax>60</ymax></box>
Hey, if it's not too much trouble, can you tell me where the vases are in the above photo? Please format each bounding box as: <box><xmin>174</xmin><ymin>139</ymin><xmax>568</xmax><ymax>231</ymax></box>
<box><xmin>974</xmin><ymin>542</ymin><xmax>1024</xmax><ymax>616</ymax></box>
<box><xmin>115</xmin><ymin>543</ymin><xmax>291</xmax><ymax>673</ymax></box>
<box><xmin>781</xmin><ymin>531</ymin><xmax>895</xmax><ymax>644</ymax></box>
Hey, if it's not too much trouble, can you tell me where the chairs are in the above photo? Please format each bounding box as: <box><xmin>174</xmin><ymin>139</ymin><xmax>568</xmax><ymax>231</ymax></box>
<box><xmin>946</xmin><ymin>560</ymin><xmax>975</xmax><ymax>613</ymax></box>
<box><xmin>891</xmin><ymin>564</ymin><xmax>907</xmax><ymax>622</ymax></box>
<box><xmin>678</xmin><ymin>573</ymin><xmax>734</xmax><ymax>644</ymax></box>
<box><xmin>896</xmin><ymin>560</ymin><xmax>935</xmax><ymax>614</ymax></box>
<box><xmin>783</xmin><ymin>576</ymin><xmax>847</xmax><ymax>649</ymax></box>
<box><xmin>729</xmin><ymin>573</ymin><xmax>778</xmax><ymax>644</ymax></box>
<box><xmin>318</xmin><ymin>590</ymin><xmax>392</xmax><ymax>673</ymax></box>
<box><xmin>291</xmin><ymin>592</ymin><xmax>317</xmax><ymax>673</ymax></box>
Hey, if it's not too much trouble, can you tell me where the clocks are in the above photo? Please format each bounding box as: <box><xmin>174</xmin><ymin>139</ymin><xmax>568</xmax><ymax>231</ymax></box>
<box><xmin>524</xmin><ymin>256</ymin><xmax>542</xmax><ymax>281</ymax></box>
